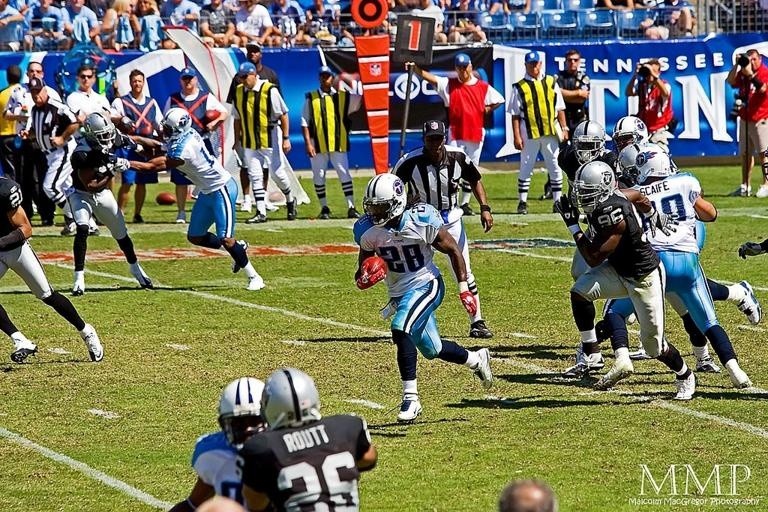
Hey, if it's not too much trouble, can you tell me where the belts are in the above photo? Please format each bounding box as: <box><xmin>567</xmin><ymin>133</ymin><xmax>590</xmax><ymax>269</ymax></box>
<box><xmin>41</xmin><ymin>137</ymin><xmax>70</xmax><ymax>155</ymax></box>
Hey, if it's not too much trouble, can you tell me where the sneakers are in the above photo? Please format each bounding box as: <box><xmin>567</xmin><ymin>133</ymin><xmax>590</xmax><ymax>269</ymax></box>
<box><xmin>83</xmin><ymin>324</ymin><xmax>103</xmax><ymax>361</ymax></box>
<box><xmin>468</xmin><ymin>321</ymin><xmax>490</xmax><ymax>338</ymax></box>
<box><xmin>232</xmin><ymin>239</ymin><xmax>264</xmax><ymax>291</ymax></box>
<box><xmin>129</xmin><ymin>267</ymin><xmax>151</xmax><ymax>288</ymax></box>
<box><xmin>461</xmin><ymin>203</ymin><xmax>474</xmax><ymax>215</ymax></box>
<box><xmin>516</xmin><ymin>201</ymin><xmax>526</xmax><ymax>213</ymax></box>
<box><xmin>736</xmin><ymin>279</ymin><xmax>761</xmax><ymax>325</ymax></box>
<box><xmin>396</xmin><ymin>392</ymin><xmax>422</xmax><ymax>421</ymax></box>
<box><xmin>134</xmin><ymin>215</ymin><xmax>142</xmax><ymax>223</ymax></box>
<box><xmin>30</xmin><ymin>218</ymin><xmax>104</xmax><ymax>236</ymax></box>
<box><xmin>73</xmin><ymin>278</ymin><xmax>85</xmax><ymax>295</ymax></box>
<box><xmin>473</xmin><ymin>348</ymin><xmax>491</xmax><ymax>389</ymax></box>
<box><xmin>239</xmin><ymin>197</ymin><xmax>361</xmax><ymax>224</ymax></box>
<box><xmin>730</xmin><ymin>183</ymin><xmax>768</xmax><ymax>198</ymax></box>
<box><xmin>176</xmin><ymin>209</ymin><xmax>184</xmax><ymax>223</ymax></box>
<box><xmin>10</xmin><ymin>341</ymin><xmax>38</xmax><ymax>362</ymax></box>
<box><xmin>562</xmin><ymin>348</ymin><xmax>751</xmax><ymax>401</ymax></box>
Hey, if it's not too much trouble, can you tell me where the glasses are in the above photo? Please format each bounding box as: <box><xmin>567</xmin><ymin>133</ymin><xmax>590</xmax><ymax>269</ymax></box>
<box><xmin>79</xmin><ymin>75</ymin><xmax>92</xmax><ymax>79</ymax></box>
<box><xmin>567</xmin><ymin>57</ymin><xmax>578</xmax><ymax>62</ymax></box>
<box><xmin>28</xmin><ymin>69</ymin><xmax>43</xmax><ymax>74</ymax></box>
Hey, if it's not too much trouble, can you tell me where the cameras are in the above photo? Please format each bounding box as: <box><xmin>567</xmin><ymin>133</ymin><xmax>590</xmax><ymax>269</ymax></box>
<box><xmin>638</xmin><ymin>64</ymin><xmax>651</xmax><ymax>76</ymax></box>
<box><xmin>737</xmin><ymin>53</ymin><xmax>751</xmax><ymax>69</ymax></box>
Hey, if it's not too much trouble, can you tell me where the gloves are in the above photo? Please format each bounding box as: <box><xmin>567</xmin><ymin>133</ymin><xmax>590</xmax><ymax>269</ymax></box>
<box><xmin>647</xmin><ymin>211</ymin><xmax>680</xmax><ymax>238</ymax></box>
<box><xmin>356</xmin><ymin>269</ymin><xmax>385</xmax><ymax>290</ymax></box>
<box><xmin>738</xmin><ymin>242</ymin><xmax>760</xmax><ymax>260</ymax></box>
<box><xmin>554</xmin><ymin>195</ymin><xmax>579</xmax><ymax>228</ymax></box>
<box><xmin>457</xmin><ymin>291</ymin><xmax>477</xmax><ymax>316</ymax></box>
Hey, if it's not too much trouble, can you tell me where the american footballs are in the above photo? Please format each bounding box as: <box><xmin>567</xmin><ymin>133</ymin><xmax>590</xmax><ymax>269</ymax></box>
<box><xmin>362</xmin><ymin>257</ymin><xmax>387</xmax><ymax>276</ymax></box>
<box><xmin>206</xmin><ymin>111</ymin><xmax>221</xmax><ymax>123</ymax></box>
<box><xmin>156</xmin><ymin>193</ymin><xmax>176</xmax><ymax>206</ymax></box>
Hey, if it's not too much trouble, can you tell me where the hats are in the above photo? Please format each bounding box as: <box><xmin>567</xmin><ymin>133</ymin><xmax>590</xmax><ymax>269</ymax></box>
<box><xmin>245</xmin><ymin>40</ymin><xmax>260</xmax><ymax>47</ymax></box>
<box><xmin>453</xmin><ymin>53</ymin><xmax>469</xmax><ymax>65</ymax></box>
<box><xmin>319</xmin><ymin>65</ymin><xmax>331</xmax><ymax>74</ymax></box>
<box><xmin>423</xmin><ymin>120</ymin><xmax>445</xmax><ymax>137</ymax></box>
<box><xmin>180</xmin><ymin>67</ymin><xmax>195</xmax><ymax>77</ymax></box>
<box><xmin>28</xmin><ymin>77</ymin><xmax>43</xmax><ymax>88</ymax></box>
<box><xmin>236</xmin><ymin>63</ymin><xmax>255</xmax><ymax>77</ymax></box>
<box><xmin>524</xmin><ymin>51</ymin><xmax>538</xmax><ymax>63</ymax></box>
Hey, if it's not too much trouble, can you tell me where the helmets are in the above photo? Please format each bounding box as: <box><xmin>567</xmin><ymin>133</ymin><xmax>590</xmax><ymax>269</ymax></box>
<box><xmin>84</xmin><ymin>112</ymin><xmax>115</xmax><ymax>150</ymax></box>
<box><xmin>219</xmin><ymin>370</ymin><xmax>320</xmax><ymax>447</ymax></box>
<box><xmin>572</xmin><ymin>116</ymin><xmax>671</xmax><ymax>203</ymax></box>
<box><xmin>159</xmin><ymin>107</ymin><xmax>192</xmax><ymax>141</ymax></box>
<box><xmin>362</xmin><ymin>174</ymin><xmax>407</xmax><ymax>220</ymax></box>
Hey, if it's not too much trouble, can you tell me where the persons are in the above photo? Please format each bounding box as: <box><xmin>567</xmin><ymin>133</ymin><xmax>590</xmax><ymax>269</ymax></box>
<box><xmin>196</xmin><ymin>494</ymin><xmax>247</xmax><ymax>512</ymax></box>
<box><xmin>166</xmin><ymin>373</ymin><xmax>274</xmax><ymax>511</ymax></box>
<box><xmin>0</xmin><ymin>0</ymin><xmax>768</xmax><ymax>406</ymax></box>
<box><xmin>495</xmin><ymin>476</ymin><xmax>556</xmax><ymax>511</ymax></box>
<box><xmin>2</xmin><ymin>173</ymin><xmax>106</xmax><ymax>364</ymax></box>
<box><xmin>242</xmin><ymin>364</ymin><xmax>379</xmax><ymax>511</ymax></box>
<box><xmin>349</xmin><ymin>172</ymin><xmax>494</xmax><ymax>422</ymax></box>
